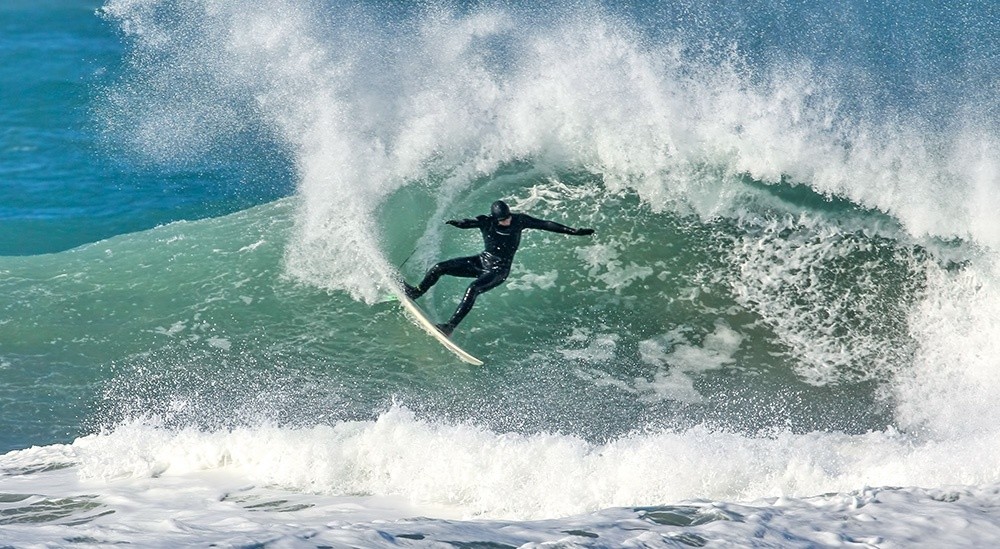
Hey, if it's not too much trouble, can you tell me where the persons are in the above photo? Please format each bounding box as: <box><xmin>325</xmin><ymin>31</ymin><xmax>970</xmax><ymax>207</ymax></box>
<box><xmin>396</xmin><ymin>201</ymin><xmax>596</xmax><ymax>337</ymax></box>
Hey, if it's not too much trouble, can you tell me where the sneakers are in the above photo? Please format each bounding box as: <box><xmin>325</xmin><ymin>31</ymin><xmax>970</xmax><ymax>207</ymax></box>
<box><xmin>435</xmin><ymin>324</ymin><xmax>452</xmax><ymax>337</ymax></box>
<box><xmin>401</xmin><ymin>280</ymin><xmax>416</xmax><ymax>301</ymax></box>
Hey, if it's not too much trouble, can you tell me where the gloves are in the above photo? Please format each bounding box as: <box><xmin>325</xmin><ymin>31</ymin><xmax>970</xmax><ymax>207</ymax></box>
<box><xmin>445</xmin><ymin>218</ymin><xmax>465</xmax><ymax>228</ymax></box>
<box><xmin>574</xmin><ymin>228</ymin><xmax>594</xmax><ymax>236</ymax></box>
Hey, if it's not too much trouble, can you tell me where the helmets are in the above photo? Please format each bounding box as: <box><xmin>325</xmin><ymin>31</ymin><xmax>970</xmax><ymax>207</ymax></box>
<box><xmin>491</xmin><ymin>200</ymin><xmax>509</xmax><ymax>221</ymax></box>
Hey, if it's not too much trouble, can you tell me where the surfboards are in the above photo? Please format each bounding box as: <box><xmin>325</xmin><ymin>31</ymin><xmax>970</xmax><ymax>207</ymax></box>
<box><xmin>385</xmin><ymin>276</ymin><xmax>485</xmax><ymax>368</ymax></box>
